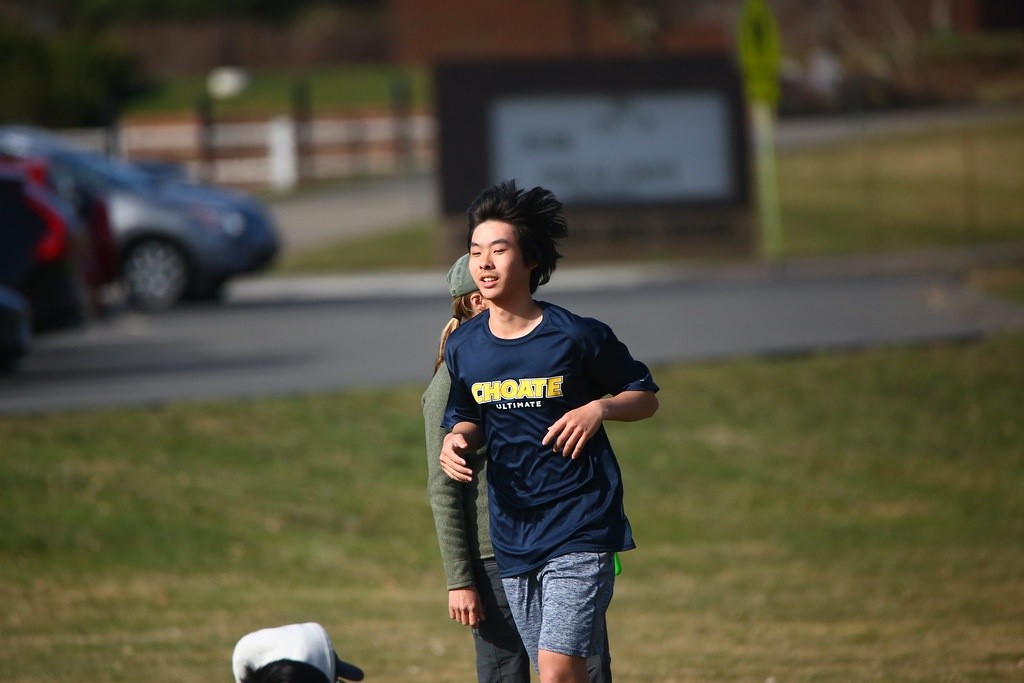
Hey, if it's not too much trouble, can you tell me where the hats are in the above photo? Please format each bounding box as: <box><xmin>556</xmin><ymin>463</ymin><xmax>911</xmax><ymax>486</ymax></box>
<box><xmin>446</xmin><ymin>253</ymin><xmax>479</xmax><ymax>298</ymax></box>
<box><xmin>231</xmin><ymin>621</ymin><xmax>364</xmax><ymax>683</ymax></box>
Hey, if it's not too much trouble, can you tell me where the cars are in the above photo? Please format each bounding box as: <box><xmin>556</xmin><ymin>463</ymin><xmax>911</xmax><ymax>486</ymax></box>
<box><xmin>0</xmin><ymin>123</ymin><xmax>286</xmax><ymax>380</ymax></box>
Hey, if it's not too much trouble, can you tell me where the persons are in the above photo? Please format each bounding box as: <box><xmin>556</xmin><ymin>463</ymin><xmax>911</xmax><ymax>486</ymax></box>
<box><xmin>423</xmin><ymin>181</ymin><xmax>660</xmax><ymax>683</ymax></box>
<box><xmin>231</xmin><ymin>621</ymin><xmax>365</xmax><ymax>683</ymax></box>
